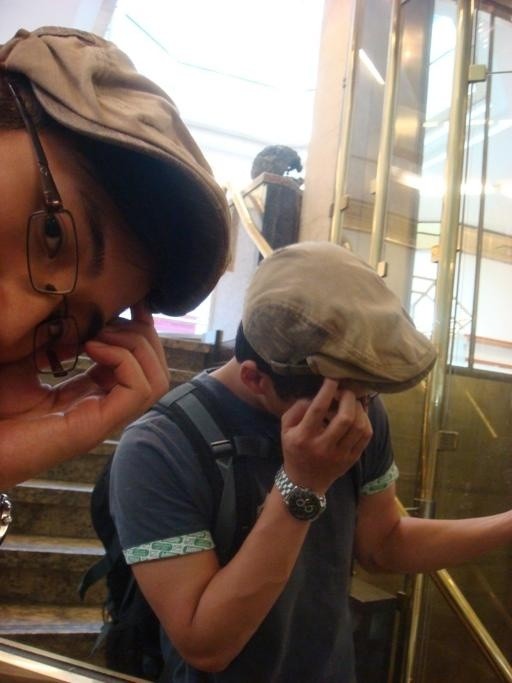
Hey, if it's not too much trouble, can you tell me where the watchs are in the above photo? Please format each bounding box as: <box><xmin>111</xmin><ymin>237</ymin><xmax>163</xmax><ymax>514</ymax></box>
<box><xmin>274</xmin><ymin>461</ymin><xmax>328</xmax><ymax>525</ymax></box>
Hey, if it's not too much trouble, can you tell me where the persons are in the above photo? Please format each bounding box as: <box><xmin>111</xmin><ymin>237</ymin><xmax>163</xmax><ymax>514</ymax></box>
<box><xmin>1</xmin><ymin>24</ymin><xmax>234</xmax><ymax>500</ymax></box>
<box><xmin>87</xmin><ymin>238</ymin><xmax>511</xmax><ymax>683</ymax></box>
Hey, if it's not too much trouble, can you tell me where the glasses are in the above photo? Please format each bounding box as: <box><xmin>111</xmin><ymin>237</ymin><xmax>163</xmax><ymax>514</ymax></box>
<box><xmin>5</xmin><ymin>74</ymin><xmax>82</xmax><ymax>378</ymax></box>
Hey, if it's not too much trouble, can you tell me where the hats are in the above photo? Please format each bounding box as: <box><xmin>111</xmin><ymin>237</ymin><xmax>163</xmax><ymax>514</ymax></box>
<box><xmin>1</xmin><ymin>24</ymin><xmax>233</xmax><ymax>318</ymax></box>
<box><xmin>241</xmin><ymin>238</ymin><xmax>439</xmax><ymax>394</ymax></box>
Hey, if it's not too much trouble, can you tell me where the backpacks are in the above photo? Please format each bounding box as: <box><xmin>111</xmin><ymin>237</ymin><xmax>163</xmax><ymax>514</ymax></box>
<box><xmin>76</xmin><ymin>378</ymin><xmax>244</xmax><ymax>683</ymax></box>
